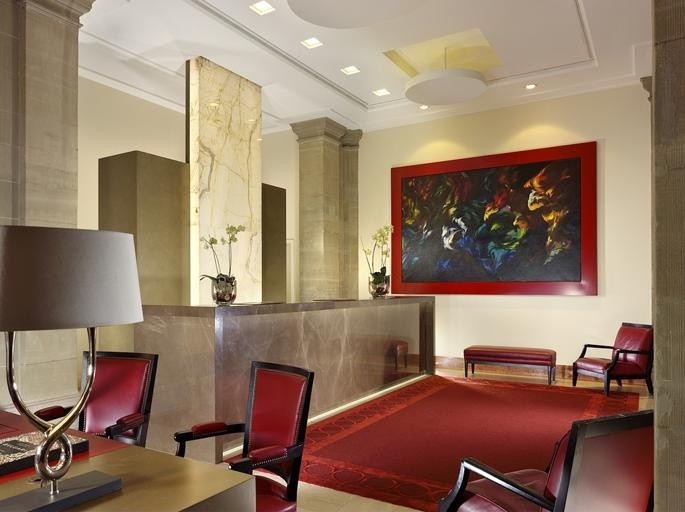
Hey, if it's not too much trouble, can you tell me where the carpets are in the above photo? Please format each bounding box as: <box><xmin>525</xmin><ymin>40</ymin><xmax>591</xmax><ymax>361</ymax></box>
<box><xmin>223</xmin><ymin>375</ymin><xmax>639</xmax><ymax>512</ymax></box>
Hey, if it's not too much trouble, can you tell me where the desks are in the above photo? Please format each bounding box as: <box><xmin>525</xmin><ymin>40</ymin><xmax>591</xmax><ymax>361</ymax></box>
<box><xmin>0</xmin><ymin>410</ymin><xmax>256</xmax><ymax>512</ymax></box>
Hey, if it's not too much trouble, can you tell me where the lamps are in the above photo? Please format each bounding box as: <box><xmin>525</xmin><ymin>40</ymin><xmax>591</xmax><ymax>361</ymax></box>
<box><xmin>0</xmin><ymin>224</ymin><xmax>144</xmax><ymax>512</ymax></box>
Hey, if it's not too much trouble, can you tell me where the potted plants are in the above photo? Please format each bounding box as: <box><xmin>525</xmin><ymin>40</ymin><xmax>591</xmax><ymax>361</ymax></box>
<box><xmin>360</xmin><ymin>224</ymin><xmax>394</xmax><ymax>299</ymax></box>
<box><xmin>199</xmin><ymin>224</ymin><xmax>245</xmax><ymax>305</ymax></box>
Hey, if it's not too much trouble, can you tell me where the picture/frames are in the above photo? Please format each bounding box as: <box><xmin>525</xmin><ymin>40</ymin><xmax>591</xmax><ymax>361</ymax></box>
<box><xmin>391</xmin><ymin>141</ymin><xmax>597</xmax><ymax>296</ymax></box>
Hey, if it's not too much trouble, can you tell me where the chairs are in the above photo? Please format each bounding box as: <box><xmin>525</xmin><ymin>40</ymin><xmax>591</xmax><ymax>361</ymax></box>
<box><xmin>173</xmin><ymin>361</ymin><xmax>314</xmax><ymax>512</ymax></box>
<box><xmin>436</xmin><ymin>409</ymin><xmax>653</xmax><ymax>512</ymax></box>
<box><xmin>571</xmin><ymin>323</ymin><xmax>654</xmax><ymax>395</ymax></box>
<box><xmin>34</xmin><ymin>349</ymin><xmax>158</xmax><ymax>446</ymax></box>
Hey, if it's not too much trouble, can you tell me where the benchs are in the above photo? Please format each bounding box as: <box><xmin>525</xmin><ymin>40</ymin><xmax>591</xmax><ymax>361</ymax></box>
<box><xmin>464</xmin><ymin>346</ymin><xmax>556</xmax><ymax>385</ymax></box>
<box><xmin>387</xmin><ymin>339</ymin><xmax>408</xmax><ymax>371</ymax></box>
